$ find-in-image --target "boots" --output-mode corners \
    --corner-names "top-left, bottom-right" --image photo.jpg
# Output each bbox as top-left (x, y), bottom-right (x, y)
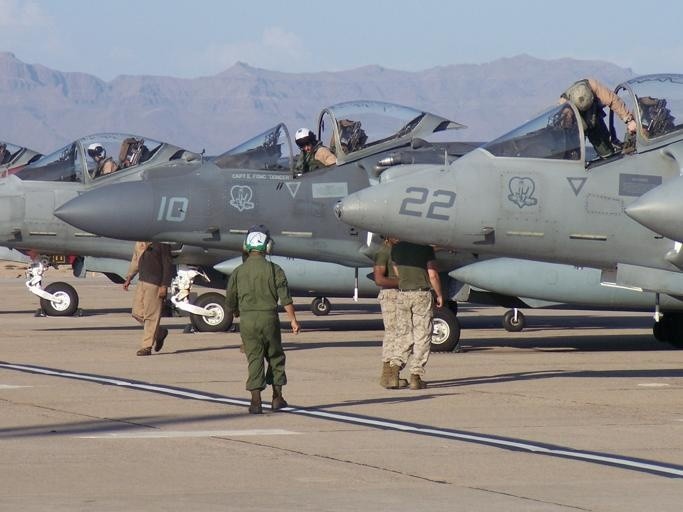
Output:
top-left (380, 363), bottom-right (426, 390)
top-left (249, 390), bottom-right (262, 414)
top-left (272, 385), bottom-right (287, 410)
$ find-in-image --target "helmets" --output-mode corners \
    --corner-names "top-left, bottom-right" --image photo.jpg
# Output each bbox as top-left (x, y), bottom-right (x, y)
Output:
top-left (294, 127), bottom-right (316, 148)
top-left (567, 83), bottom-right (594, 112)
top-left (88, 143), bottom-right (106, 159)
top-left (244, 225), bottom-right (270, 253)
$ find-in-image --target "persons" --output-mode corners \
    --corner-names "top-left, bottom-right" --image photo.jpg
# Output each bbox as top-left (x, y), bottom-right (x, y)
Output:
top-left (224, 232), bottom-right (301, 414)
top-left (386, 240), bottom-right (444, 390)
top-left (88, 142), bottom-right (120, 179)
top-left (374, 234), bottom-right (409, 389)
top-left (233, 224), bottom-right (276, 386)
top-left (560, 78), bottom-right (637, 161)
top-left (293, 127), bottom-right (337, 178)
top-left (124, 242), bottom-right (174, 356)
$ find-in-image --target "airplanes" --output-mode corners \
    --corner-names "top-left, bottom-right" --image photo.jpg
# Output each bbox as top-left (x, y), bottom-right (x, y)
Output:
top-left (0, 141), bottom-right (82, 318)
top-left (52, 97), bottom-right (527, 351)
top-left (624, 176), bottom-right (682, 270)
top-left (0, 133), bottom-right (368, 333)
top-left (333, 73), bottom-right (683, 355)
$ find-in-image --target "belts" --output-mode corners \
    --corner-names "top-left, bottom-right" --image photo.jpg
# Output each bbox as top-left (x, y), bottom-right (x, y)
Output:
top-left (399, 287), bottom-right (429, 292)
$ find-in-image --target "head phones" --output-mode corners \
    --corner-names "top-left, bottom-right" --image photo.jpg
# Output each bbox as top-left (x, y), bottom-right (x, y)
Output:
top-left (242, 240), bottom-right (273, 254)
top-left (95, 146), bottom-right (105, 153)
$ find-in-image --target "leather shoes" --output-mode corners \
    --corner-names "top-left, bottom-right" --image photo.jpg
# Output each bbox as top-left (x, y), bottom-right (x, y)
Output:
top-left (136, 348), bottom-right (151, 356)
top-left (154, 328), bottom-right (168, 351)
